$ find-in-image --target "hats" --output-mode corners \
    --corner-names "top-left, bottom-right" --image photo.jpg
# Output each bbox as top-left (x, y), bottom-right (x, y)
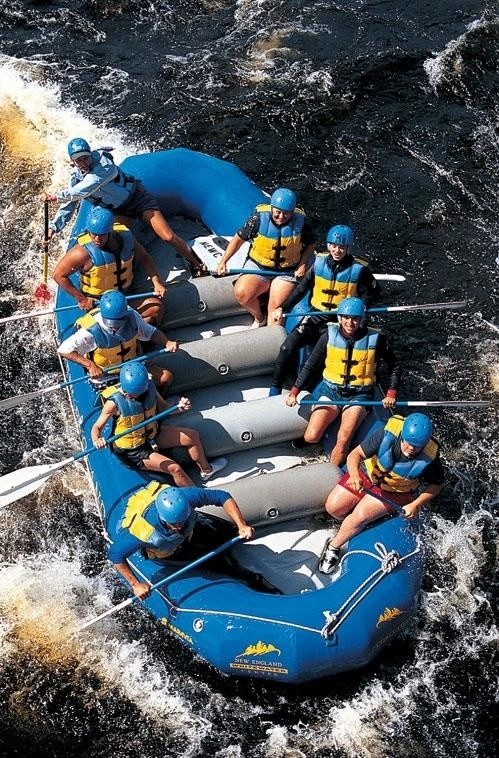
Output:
top-left (103, 316), bottom-right (126, 328)
top-left (70, 151), bottom-right (91, 160)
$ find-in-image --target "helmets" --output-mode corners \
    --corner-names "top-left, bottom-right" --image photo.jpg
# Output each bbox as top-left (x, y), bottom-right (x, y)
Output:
top-left (100, 291), bottom-right (128, 320)
top-left (68, 138), bottom-right (90, 158)
top-left (86, 208), bottom-right (113, 235)
top-left (399, 413), bottom-right (432, 448)
top-left (155, 486), bottom-right (191, 523)
top-left (326, 224), bottom-right (355, 255)
top-left (337, 297), bottom-right (367, 325)
top-left (119, 362), bottom-right (149, 395)
top-left (271, 188), bottom-right (296, 214)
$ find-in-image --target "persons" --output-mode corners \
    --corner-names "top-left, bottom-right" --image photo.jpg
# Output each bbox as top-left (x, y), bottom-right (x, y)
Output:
top-left (57, 290), bottom-right (178, 398)
top-left (287, 298), bottom-right (402, 466)
top-left (40, 138), bottom-right (207, 276)
top-left (107, 480), bottom-right (284, 602)
top-left (269, 225), bottom-right (382, 395)
top-left (216, 189), bottom-right (317, 329)
top-left (91, 362), bottom-right (227, 488)
top-left (319, 412), bottom-right (445, 574)
top-left (53, 209), bottom-right (168, 327)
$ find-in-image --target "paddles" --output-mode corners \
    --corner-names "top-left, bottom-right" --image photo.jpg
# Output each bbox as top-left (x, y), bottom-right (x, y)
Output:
top-left (281, 301), bottom-right (467, 317)
top-left (0, 400), bottom-right (186, 508)
top-left (210, 269), bottom-right (406, 282)
top-left (0, 292), bottom-right (156, 325)
top-left (33, 200), bottom-right (50, 301)
top-left (79, 532), bottom-right (246, 631)
top-left (0, 347), bottom-right (169, 412)
top-left (297, 401), bottom-right (490, 408)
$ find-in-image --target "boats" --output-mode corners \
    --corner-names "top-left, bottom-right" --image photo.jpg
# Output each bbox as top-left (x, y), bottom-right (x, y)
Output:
top-left (54, 147), bottom-right (425, 686)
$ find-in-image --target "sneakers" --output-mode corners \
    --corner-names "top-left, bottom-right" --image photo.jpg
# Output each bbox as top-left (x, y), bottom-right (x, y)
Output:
top-left (199, 458), bottom-right (228, 481)
top-left (319, 537), bottom-right (340, 575)
top-left (313, 511), bottom-right (342, 525)
top-left (247, 573), bottom-right (276, 594)
top-left (291, 437), bottom-right (307, 449)
top-left (249, 315), bottom-right (267, 329)
